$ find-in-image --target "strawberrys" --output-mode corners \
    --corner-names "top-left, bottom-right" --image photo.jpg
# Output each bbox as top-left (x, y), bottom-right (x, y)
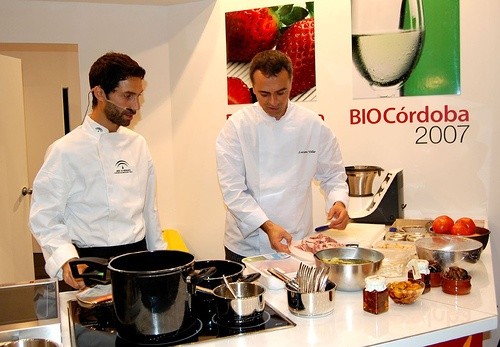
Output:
top-left (225, 1), bottom-right (316, 105)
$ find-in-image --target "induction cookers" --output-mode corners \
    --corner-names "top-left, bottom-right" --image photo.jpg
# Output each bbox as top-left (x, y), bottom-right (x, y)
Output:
top-left (59, 272), bottom-right (296, 346)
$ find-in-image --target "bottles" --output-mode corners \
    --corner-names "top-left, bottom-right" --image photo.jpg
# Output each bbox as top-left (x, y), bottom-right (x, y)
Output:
top-left (363, 275), bottom-right (389, 314)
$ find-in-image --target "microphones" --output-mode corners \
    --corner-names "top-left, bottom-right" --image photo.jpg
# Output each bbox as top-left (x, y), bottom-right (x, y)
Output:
top-left (105, 99), bottom-right (133, 112)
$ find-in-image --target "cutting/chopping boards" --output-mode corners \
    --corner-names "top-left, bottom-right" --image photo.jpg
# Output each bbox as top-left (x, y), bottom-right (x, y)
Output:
top-left (372, 218), bottom-right (484, 248)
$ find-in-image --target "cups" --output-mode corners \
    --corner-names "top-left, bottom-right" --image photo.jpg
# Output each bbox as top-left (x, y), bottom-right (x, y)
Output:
top-left (441, 274), bottom-right (471, 295)
top-left (408, 260), bottom-right (431, 295)
top-left (425, 270), bottom-right (446, 288)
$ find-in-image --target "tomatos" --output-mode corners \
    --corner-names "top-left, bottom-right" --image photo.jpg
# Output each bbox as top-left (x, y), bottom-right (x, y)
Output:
top-left (432, 216), bottom-right (479, 237)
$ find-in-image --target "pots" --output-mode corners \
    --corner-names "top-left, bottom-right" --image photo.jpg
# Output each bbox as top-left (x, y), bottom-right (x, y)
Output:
top-left (69, 250), bottom-right (195, 342)
top-left (194, 260), bottom-right (244, 289)
top-left (313, 244), bottom-right (384, 292)
top-left (194, 282), bottom-right (266, 324)
top-left (344, 166), bottom-right (384, 197)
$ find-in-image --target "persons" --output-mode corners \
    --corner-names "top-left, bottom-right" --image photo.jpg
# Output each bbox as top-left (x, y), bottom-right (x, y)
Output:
top-left (28, 52), bottom-right (167, 292)
top-left (215, 51), bottom-right (349, 268)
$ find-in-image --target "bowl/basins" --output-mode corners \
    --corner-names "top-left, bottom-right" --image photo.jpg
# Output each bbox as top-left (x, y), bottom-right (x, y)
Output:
top-left (428, 226), bottom-right (491, 249)
top-left (416, 237), bottom-right (483, 275)
top-left (387, 278), bottom-right (426, 305)
top-left (284, 277), bottom-right (336, 319)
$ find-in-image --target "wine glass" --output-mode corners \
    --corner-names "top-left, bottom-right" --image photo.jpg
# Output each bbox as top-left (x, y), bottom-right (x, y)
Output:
top-left (351, 0), bottom-right (425, 98)
